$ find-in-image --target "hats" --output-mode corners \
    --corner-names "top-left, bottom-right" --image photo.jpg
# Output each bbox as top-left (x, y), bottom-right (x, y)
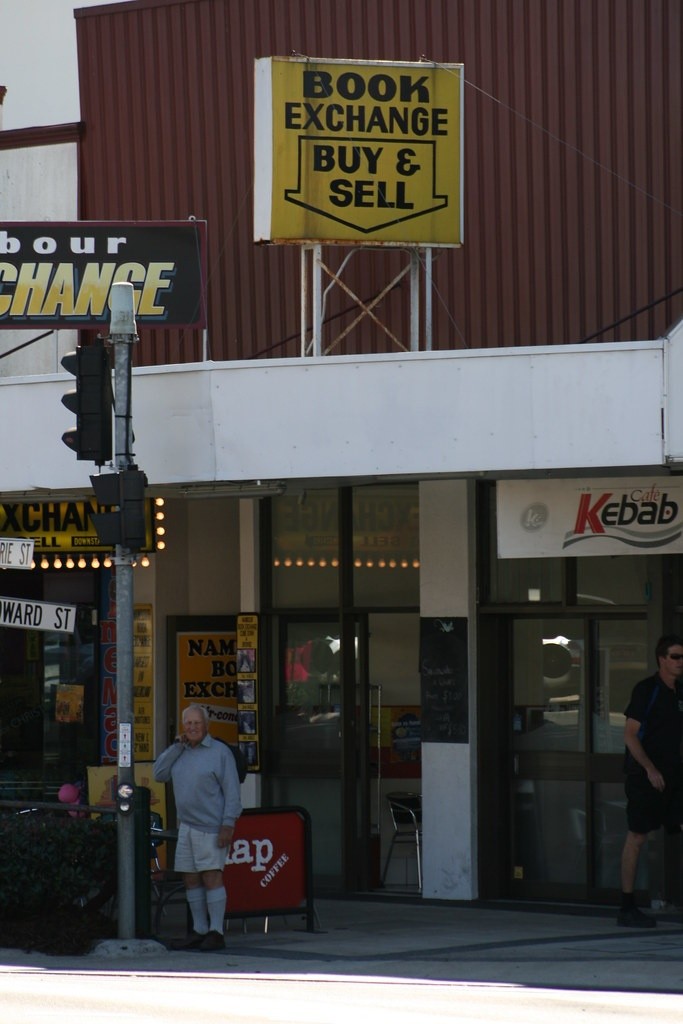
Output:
top-left (58, 783), bottom-right (81, 802)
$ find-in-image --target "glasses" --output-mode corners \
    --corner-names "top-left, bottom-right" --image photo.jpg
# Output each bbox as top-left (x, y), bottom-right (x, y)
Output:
top-left (670, 653), bottom-right (683, 660)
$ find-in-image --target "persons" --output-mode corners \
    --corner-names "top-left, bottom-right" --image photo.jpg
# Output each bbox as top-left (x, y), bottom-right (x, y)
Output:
top-left (617, 633), bottom-right (683, 927)
top-left (153, 703), bottom-right (242, 951)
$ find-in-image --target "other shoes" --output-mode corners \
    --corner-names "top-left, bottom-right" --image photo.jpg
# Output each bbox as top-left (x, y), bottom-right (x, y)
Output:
top-left (198, 930), bottom-right (226, 951)
top-left (617, 907), bottom-right (657, 927)
top-left (171, 938), bottom-right (199, 949)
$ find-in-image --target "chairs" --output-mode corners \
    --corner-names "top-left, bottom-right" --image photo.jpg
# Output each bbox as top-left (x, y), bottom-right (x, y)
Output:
top-left (380, 791), bottom-right (422, 892)
top-left (98, 809), bottom-right (167, 920)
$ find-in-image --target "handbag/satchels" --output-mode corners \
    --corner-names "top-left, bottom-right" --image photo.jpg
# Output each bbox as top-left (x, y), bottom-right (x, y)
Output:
top-left (625, 684), bottom-right (660, 762)
